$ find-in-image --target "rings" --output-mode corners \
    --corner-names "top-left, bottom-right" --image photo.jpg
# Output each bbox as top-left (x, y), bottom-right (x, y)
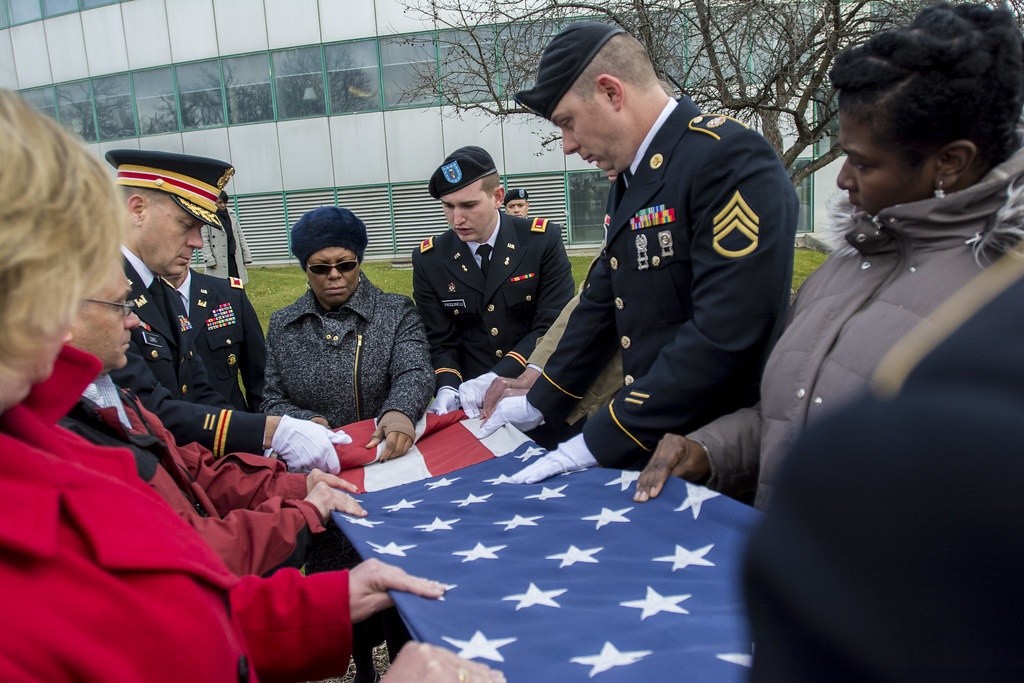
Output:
top-left (458, 666), bottom-right (472, 683)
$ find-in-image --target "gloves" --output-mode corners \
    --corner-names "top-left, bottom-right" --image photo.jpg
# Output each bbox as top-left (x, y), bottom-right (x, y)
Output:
top-left (458, 371), bottom-right (499, 419)
top-left (427, 386), bottom-right (463, 416)
top-left (475, 395), bottom-right (543, 439)
top-left (271, 415), bottom-right (353, 474)
top-left (509, 432), bottom-right (599, 486)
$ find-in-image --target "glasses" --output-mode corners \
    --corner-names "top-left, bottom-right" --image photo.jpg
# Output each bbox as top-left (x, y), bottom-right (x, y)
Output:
top-left (307, 254), bottom-right (358, 276)
top-left (85, 298), bottom-right (135, 317)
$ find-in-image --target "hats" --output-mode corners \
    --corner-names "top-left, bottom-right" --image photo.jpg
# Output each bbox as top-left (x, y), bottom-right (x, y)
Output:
top-left (512, 21), bottom-right (627, 119)
top-left (105, 149), bottom-right (236, 231)
top-left (503, 188), bottom-right (528, 206)
top-left (428, 145), bottom-right (498, 199)
top-left (291, 205), bottom-right (369, 271)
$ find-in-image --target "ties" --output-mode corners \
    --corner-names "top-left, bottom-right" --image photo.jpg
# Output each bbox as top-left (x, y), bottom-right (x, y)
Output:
top-left (614, 166), bottom-right (634, 218)
top-left (173, 291), bottom-right (189, 323)
top-left (475, 244), bottom-right (492, 277)
top-left (148, 278), bottom-right (171, 332)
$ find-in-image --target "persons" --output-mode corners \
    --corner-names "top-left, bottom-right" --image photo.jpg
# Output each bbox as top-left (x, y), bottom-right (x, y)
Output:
top-left (478, 21), bottom-right (801, 484)
top-left (412, 144), bottom-right (574, 420)
top-left (481, 162), bottom-right (625, 453)
top-left (637, 4), bottom-right (1024, 683)
top-left (0, 86), bottom-right (507, 683)
top-left (502, 188), bottom-right (529, 219)
